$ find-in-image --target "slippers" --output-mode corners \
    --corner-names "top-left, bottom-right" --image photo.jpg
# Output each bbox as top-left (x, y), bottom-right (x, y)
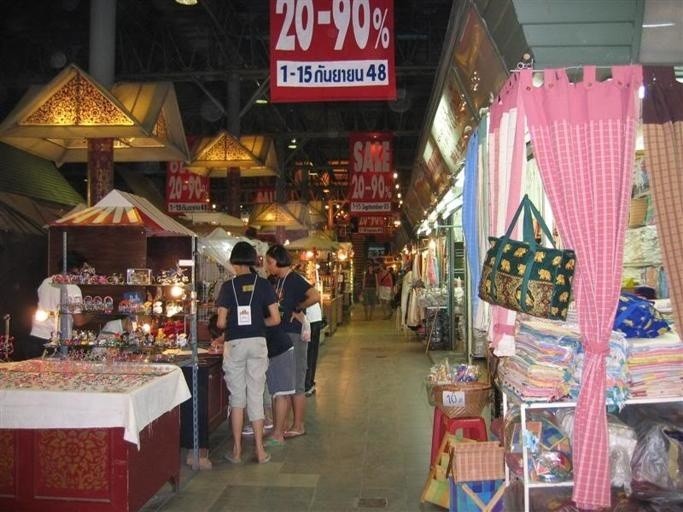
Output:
top-left (261, 433), bottom-right (286, 447)
top-left (257, 450), bottom-right (271, 464)
top-left (222, 450), bottom-right (243, 465)
top-left (283, 428), bottom-right (305, 438)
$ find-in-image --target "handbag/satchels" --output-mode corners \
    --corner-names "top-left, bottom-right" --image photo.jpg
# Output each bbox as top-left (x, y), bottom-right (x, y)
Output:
top-left (612, 292), bottom-right (672, 339)
top-left (477, 235), bottom-right (577, 321)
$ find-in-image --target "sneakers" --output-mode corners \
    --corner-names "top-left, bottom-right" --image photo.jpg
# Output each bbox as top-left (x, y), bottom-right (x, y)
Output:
top-left (305, 384), bottom-right (315, 397)
top-left (261, 416), bottom-right (274, 428)
top-left (241, 423), bottom-right (265, 435)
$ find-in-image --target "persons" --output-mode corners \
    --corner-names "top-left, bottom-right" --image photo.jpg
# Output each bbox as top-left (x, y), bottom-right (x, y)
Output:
top-left (95, 313), bottom-right (136, 341)
top-left (360, 262), bottom-right (405, 322)
top-left (217, 241), bottom-right (280, 464)
top-left (209, 266), bottom-right (322, 449)
top-left (266, 246), bottom-right (320, 439)
top-left (29, 250), bottom-right (91, 360)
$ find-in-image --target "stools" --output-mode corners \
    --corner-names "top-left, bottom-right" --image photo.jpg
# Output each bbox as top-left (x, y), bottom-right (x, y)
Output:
top-left (439, 413), bottom-right (489, 452)
top-left (432, 406), bottom-right (447, 463)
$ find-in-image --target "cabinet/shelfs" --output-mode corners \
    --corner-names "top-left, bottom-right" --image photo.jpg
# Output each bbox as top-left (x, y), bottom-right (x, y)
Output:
top-left (50, 262), bottom-right (196, 367)
top-left (0, 359), bottom-right (192, 511)
top-left (180, 354), bottom-right (231, 452)
top-left (502, 389), bottom-right (683, 512)
